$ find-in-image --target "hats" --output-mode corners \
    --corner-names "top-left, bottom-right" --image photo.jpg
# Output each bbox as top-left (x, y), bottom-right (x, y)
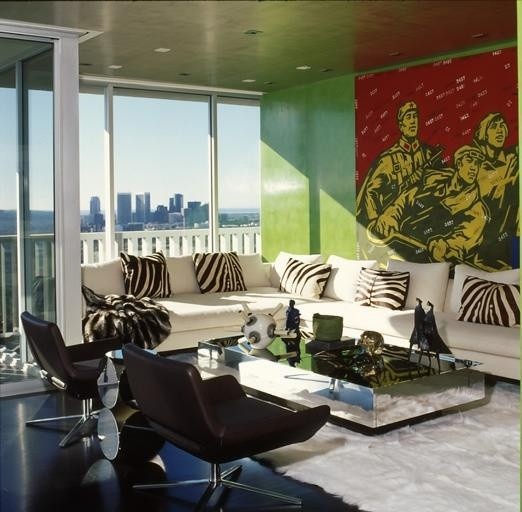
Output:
top-left (454, 145), bottom-right (482, 164)
top-left (398, 101), bottom-right (417, 121)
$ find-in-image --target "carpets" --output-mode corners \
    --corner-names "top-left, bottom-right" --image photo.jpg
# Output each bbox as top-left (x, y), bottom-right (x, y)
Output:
top-left (161, 345), bottom-right (521, 511)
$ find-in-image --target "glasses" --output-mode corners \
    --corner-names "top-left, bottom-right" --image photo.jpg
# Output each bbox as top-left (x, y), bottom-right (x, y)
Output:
top-left (459, 150), bottom-right (486, 161)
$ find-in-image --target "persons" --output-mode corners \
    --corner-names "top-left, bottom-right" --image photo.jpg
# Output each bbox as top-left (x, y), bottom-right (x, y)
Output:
top-left (356, 101), bottom-right (448, 260)
top-left (375, 144), bottom-right (492, 279)
top-left (471, 112), bottom-right (519, 272)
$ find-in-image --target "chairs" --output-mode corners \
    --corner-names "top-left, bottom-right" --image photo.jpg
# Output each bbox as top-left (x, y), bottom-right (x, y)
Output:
top-left (121, 342), bottom-right (331, 512)
top-left (21, 311), bottom-right (124, 448)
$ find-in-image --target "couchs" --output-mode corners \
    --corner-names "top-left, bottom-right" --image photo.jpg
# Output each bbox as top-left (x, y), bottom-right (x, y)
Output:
top-left (77, 251), bottom-right (522, 383)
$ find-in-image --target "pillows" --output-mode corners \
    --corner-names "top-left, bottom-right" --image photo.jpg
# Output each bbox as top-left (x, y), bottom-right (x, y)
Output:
top-left (237, 253), bottom-right (272, 287)
top-left (192, 252), bottom-right (248, 294)
top-left (324, 255), bottom-right (378, 303)
top-left (449, 264), bottom-right (520, 313)
top-left (166, 256), bottom-right (201, 295)
top-left (269, 251), bottom-right (322, 288)
top-left (81, 257), bottom-right (125, 295)
top-left (353, 267), bottom-right (409, 310)
top-left (457, 274), bottom-right (520, 328)
top-left (386, 259), bottom-right (452, 312)
top-left (120, 250), bottom-right (171, 298)
top-left (277, 257), bottom-right (332, 299)
